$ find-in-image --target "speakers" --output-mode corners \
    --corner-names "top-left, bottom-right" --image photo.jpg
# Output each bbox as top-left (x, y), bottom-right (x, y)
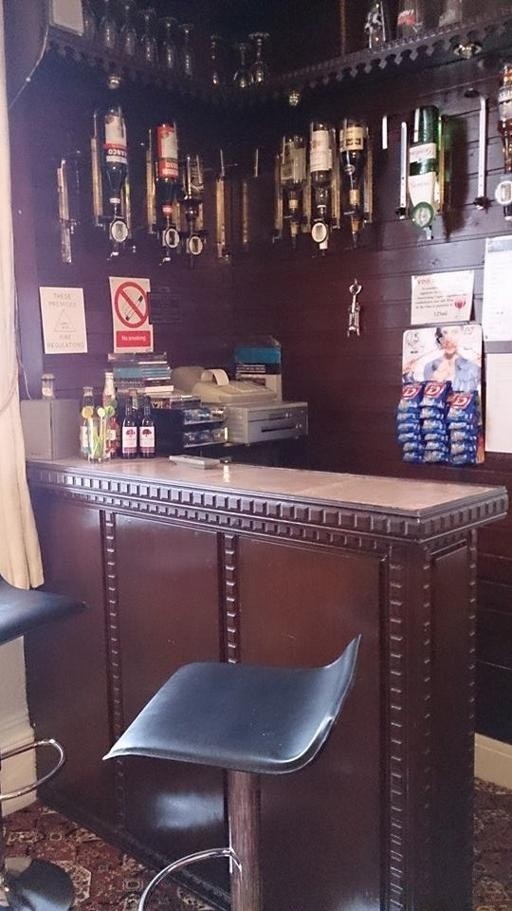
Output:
top-left (21, 398), bottom-right (81, 460)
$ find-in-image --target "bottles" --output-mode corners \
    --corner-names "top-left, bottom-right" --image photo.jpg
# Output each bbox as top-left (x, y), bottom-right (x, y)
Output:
top-left (41, 373), bottom-right (55, 400)
top-left (181, 147), bottom-right (203, 259)
top-left (149, 109), bottom-right (181, 263)
top-left (308, 117), bottom-right (331, 257)
top-left (498, 65), bottom-right (512, 222)
top-left (93, 105), bottom-right (128, 260)
top-left (280, 131), bottom-right (304, 255)
top-left (338, 115), bottom-right (365, 250)
top-left (408, 105), bottom-right (440, 242)
top-left (81, 372), bottom-right (155, 459)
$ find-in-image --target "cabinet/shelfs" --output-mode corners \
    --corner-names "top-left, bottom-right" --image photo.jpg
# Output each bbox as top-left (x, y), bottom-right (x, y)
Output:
top-left (22, 453), bottom-right (509, 911)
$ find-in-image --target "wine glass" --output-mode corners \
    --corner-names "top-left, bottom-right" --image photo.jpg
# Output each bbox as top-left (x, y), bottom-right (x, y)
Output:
top-left (80, 0), bottom-right (269, 83)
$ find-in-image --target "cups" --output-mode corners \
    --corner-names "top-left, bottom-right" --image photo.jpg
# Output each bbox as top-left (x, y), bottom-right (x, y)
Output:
top-left (356, 1), bottom-right (463, 49)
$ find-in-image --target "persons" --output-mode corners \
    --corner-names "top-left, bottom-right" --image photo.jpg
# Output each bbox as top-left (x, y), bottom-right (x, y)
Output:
top-left (422, 327), bottom-right (482, 396)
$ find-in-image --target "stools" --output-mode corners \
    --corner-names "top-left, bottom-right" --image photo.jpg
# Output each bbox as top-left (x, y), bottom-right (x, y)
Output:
top-left (100, 633), bottom-right (361, 911)
top-left (1, 565), bottom-right (91, 911)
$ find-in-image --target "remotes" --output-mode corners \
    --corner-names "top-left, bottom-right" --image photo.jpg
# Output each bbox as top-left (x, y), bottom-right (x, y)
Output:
top-left (169, 454), bottom-right (220, 469)
